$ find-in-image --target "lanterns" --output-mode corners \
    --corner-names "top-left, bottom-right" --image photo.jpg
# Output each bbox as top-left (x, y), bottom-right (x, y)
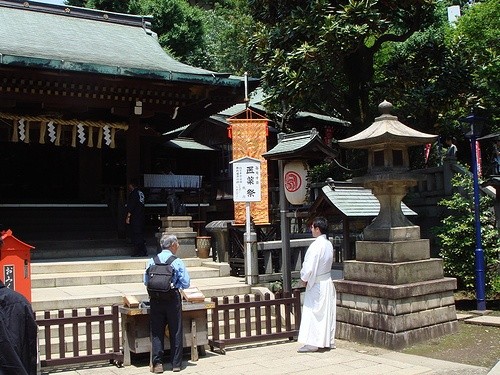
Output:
top-left (283, 160), bottom-right (310, 208)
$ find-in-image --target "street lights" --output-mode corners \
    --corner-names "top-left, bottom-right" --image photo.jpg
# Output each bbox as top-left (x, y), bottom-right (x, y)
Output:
top-left (457, 112), bottom-right (489, 312)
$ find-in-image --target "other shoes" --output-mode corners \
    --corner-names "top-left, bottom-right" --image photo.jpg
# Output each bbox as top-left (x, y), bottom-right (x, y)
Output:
top-left (297, 345), bottom-right (318, 352)
top-left (150, 363), bottom-right (163, 373)
top-left (173, 367), bottom-right (181, 372)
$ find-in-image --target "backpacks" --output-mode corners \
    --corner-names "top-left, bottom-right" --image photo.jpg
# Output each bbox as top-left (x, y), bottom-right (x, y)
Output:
top-left (146, 254), bottom-right (177, 299)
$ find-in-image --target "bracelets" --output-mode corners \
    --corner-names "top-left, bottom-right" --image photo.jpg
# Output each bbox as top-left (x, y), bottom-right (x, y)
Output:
top-left (127, 216), bottom-right (130, 218)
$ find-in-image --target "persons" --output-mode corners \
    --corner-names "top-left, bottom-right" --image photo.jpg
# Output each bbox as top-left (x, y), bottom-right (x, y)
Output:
top-left (445, 137), bottom-right (457, 158)
top-left (125, 181), bottom-right (148, 256)
top-left (0, 279), bottom-right (38, 375)
top-left (143, 234), bottom-right (190, 373)
top-left (296, 217), bottom-right (336, 352)
